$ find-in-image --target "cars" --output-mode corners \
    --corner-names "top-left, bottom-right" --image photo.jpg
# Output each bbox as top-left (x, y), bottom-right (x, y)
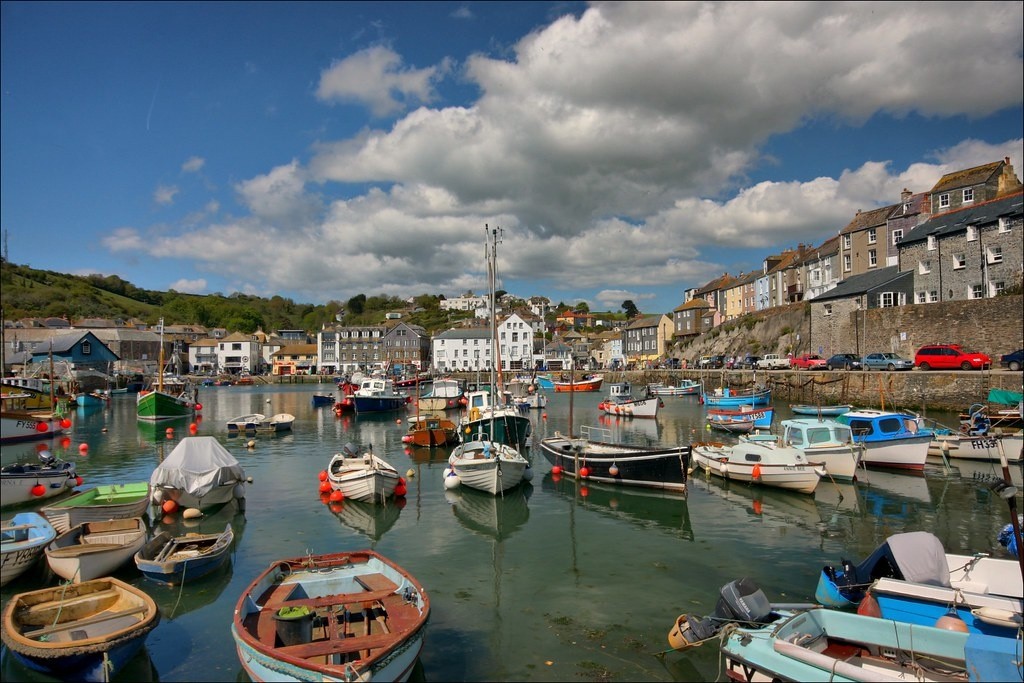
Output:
top-left (663, 343), bottom-right (1024, 372)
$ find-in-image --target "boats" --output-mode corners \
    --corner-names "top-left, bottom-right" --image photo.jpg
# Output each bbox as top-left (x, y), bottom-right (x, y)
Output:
top-left (254, 413), bottom-right (295, 432)
top-left (667, 529), bottom-right (1024, 682)
top-left (40, 481), bottom-right (151, 534)
top-left (0, 576), bottom-right (161, 683)
top-left (0, 360), bottom-right (78, 410)
top-left (94, 317), bottom-right (254, 420)
top-left (604, 414), bottom-right (664, 440)
top-left (132, 560), bottom-right (233, 621)
top-left (227, 414), bottom-right (267, 433)
top-left (0, 450), bottom-right (77, 507)
top-left (137, 420), bottom-right (190, 449)
top-left (1, 512), bottom-right (57, 586)
top-left (135, 522), bottom-right (234, 586)
top-left (151, 436), bottom-right (244, 509)
top-left (0, 393), bottom-right (65, 446)
top-left (230, 549), bottom-right (431, 683)
top-left (311, 374), bottom-right (1024, 505)
top-left (325, 454), bottom-right (1023, 542)
top-left (44, 517), bottom-right (149, 584)
top-left (76, 393), bottom-right (108, 407)
